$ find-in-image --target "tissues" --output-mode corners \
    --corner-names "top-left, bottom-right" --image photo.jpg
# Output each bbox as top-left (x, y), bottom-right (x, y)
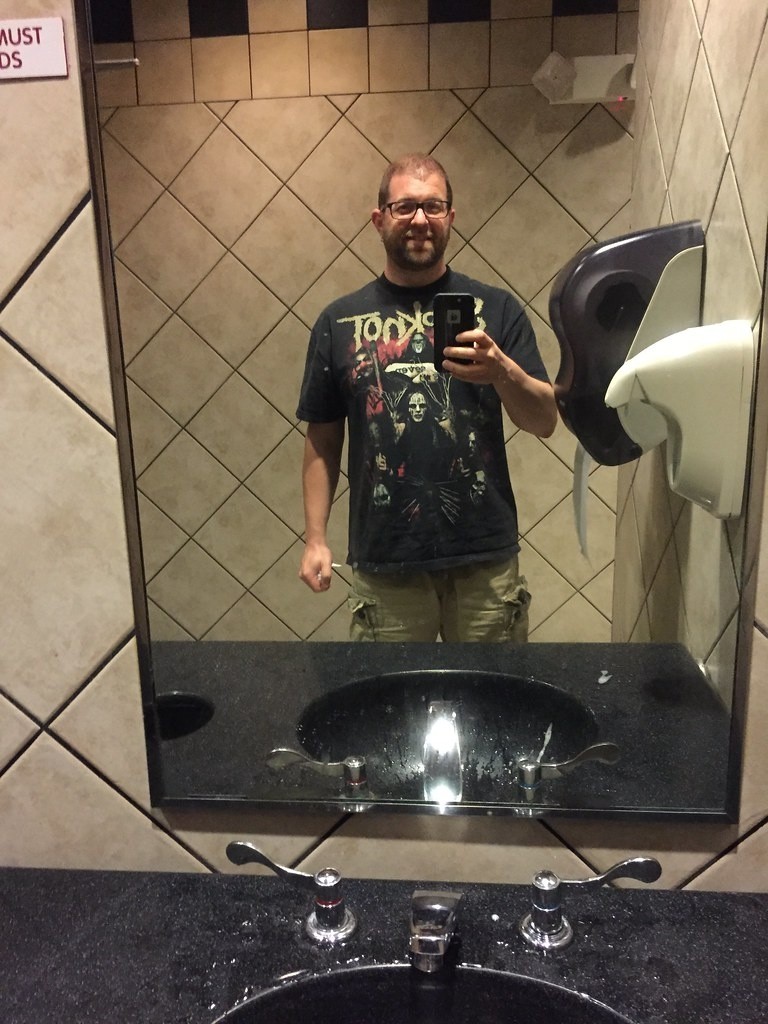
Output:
top-left (547, 221), bottom-right (704, 551)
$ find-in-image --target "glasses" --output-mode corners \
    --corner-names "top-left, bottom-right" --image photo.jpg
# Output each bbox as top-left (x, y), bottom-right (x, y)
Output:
top-left (381, 198), bottom-right (451, 220)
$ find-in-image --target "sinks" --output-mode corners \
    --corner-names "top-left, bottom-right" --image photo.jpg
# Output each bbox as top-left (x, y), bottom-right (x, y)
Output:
top-left (301, 666), bottom-right (612, 779)
top-left (205, 964), bottom-right (632, 1024)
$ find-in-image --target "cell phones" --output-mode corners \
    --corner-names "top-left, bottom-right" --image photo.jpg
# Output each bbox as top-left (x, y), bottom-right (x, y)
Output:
top-left (432, 291), bottom-right (477, 373)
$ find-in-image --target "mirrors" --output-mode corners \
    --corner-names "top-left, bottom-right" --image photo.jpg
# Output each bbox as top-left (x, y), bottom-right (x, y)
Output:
top-left (73, 0), bottom-right (768, 826)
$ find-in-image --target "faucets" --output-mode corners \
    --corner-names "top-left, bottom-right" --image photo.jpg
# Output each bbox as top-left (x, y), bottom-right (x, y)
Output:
top-left (422, 699), bottom-right (472, 808)
top-left (406, 887), bottom-right (466, 971)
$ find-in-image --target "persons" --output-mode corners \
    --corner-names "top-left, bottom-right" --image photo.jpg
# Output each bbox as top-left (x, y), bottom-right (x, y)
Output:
top-left (293, 148), bottom-right (559, 642)
